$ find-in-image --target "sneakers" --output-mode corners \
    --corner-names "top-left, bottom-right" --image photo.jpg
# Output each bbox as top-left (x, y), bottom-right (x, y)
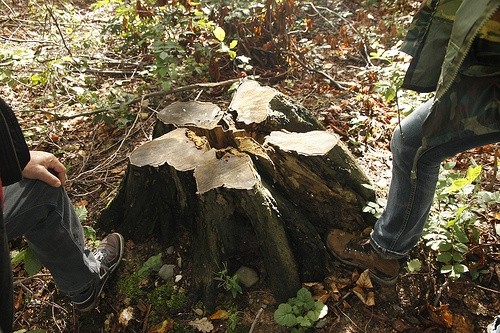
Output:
top-left (68, 232), bottom-right (124, 311)
top-left (326, 229), bottom-right (400, 287)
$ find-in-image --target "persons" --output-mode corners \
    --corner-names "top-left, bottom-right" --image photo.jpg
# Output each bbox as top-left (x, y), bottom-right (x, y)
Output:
top-left (0, 96), bottom-right (124, 333)
top-left (326, 0), bottom-right (500, 286)
